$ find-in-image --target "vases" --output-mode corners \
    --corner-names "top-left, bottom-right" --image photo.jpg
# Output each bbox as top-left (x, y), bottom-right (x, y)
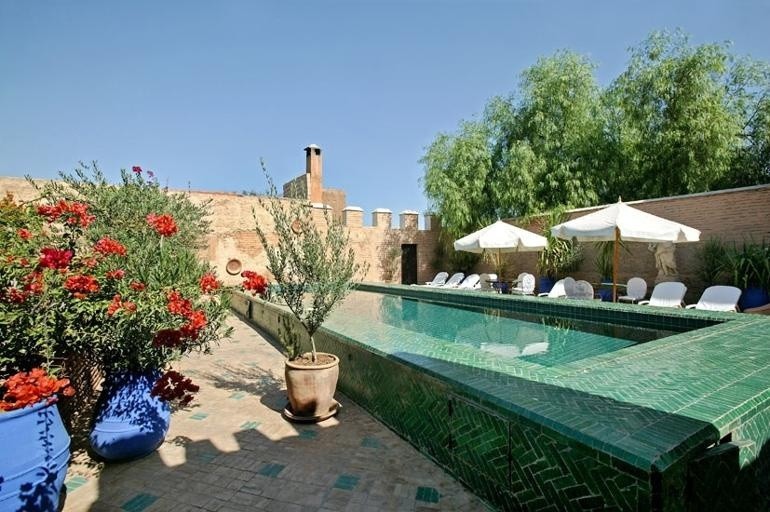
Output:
top-left (88, 361), bottom-right (170, 464)
top-left (0, 394), bottom-right (72, 512)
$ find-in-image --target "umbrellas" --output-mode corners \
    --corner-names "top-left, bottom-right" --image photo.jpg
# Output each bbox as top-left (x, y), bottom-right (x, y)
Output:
top-left (550, 194), bottom-right (703, 303)
top-left (452, 216), bottom-right (550, 282)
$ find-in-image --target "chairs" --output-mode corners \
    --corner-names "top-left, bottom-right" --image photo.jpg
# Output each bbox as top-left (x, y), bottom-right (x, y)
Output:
top-left (617, 277), bottom-right (649, 304)
top-left (510, 273), bottom-right (535, 296)
top-left (411, 272), bottom-right (497, 291)
top-left (685, 283), bottom-right (741, 313)
top-left (537, 277), bottom-right (598, 300)
top-left (638, 281), bottom-right (688, 311)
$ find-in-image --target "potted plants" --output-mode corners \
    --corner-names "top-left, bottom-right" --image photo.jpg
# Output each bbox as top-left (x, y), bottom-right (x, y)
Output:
top-left (493, 251), bottom-right (509, 293)
top-left (591, 241), bottom-right (631, 301)
top-left (536, 204), bottom-right (585, 296)
top-left (250, 158), bottom-right (368, 425)
top-left (737, 232), bottom-right (770, 312)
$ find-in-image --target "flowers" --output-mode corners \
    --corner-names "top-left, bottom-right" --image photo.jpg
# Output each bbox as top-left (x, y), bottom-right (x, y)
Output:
top-left (0, 160), bottom-right (267, 413)
